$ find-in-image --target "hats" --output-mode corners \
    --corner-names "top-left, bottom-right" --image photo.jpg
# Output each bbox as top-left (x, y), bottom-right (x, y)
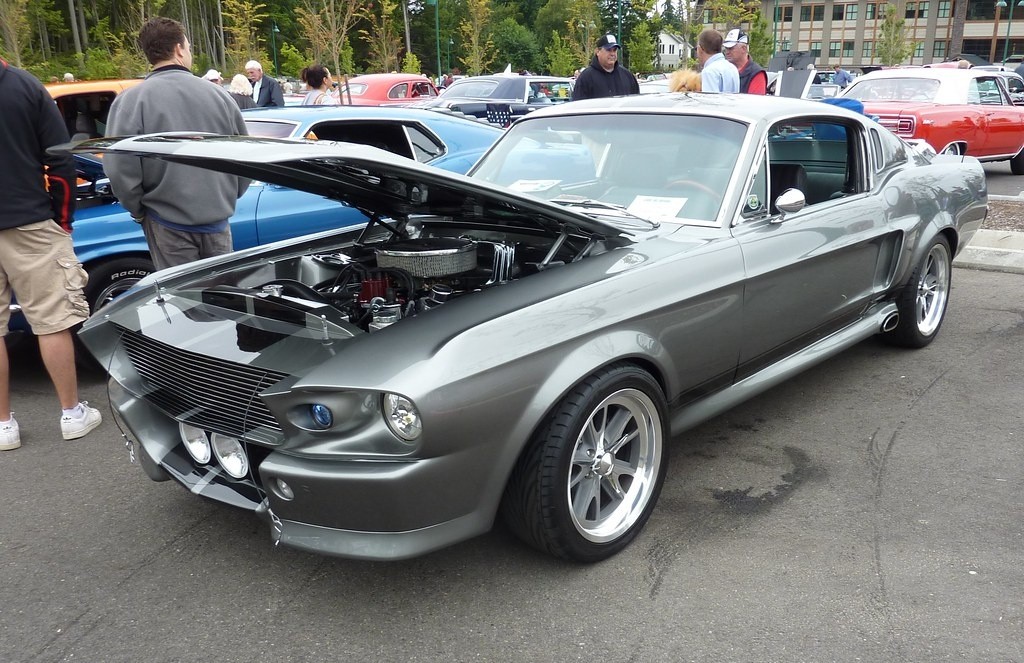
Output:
top-left (206, 70), bottom-right (220, 80)
top-left (723, 29), bottom-right (750, 47)
top-left (63, 73), bottom-right (74, 79)
top-left (597, 35), bottom-right (621, 49)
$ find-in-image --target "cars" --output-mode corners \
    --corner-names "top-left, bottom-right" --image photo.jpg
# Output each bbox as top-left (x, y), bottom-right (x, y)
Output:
top-left (637, 79), bottom-right (670, 94)
top-left (330, 74), bottom-right (440, 108)
top-left (377, 76), bottom-right (575, 127)
top-left (41, 80), bottom-right (145, 185)
top-left (8, 104), bottom-right (595, 352)
top-left (817, 71), bottom-right (837, 85)
top-left (775, 70), bottom-right (841, 101)
top-left (777, 68), bottom-right (1024, 175)
top-left (435, 75), bottom-right (465, 86)
top-left (47, 89), bottom-right (988, 565)
top-left (971, 65), bottom-right (1006, 86)
top-left (967, 73), bottom-right (1024, 106)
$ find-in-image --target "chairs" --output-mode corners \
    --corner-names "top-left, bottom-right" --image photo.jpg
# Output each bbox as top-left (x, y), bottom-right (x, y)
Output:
top-left (71, 114), bottom-right (101, 143)
top-left (769, 164), bottom-right (807, 216)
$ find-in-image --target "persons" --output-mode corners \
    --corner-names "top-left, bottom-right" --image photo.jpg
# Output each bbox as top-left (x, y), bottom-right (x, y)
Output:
top-left (807, 64), bottom-right (825, 99)
top-left (103, 17), bottom-right (252, 271)
top-left (227, 74), bottom-right (260, 110)
top-left (518, 69), bottom-right (532, 76)
top-left (51, 76), bottom-right (60, 84)
top-left (302, 65), bottom-right (340, 105)
top-left (63, 73), bottom-right (73, 83)
top-left (0, 56), bottom-right (101, 450)
top-left (696, 29), bottom-right (739, 92)
top-left (441, 73), bottom-right (453, 86)
top-left (572, 34), bottom-right (640, 102)
top-left (284, 79), bottom-right (292, 94)
top-left (201, 69), bottom-right (224, 87)
top-left (1012, 57), bottom-right (1024, 90)
top-left (723, 29), bottom-right (768, 95)
top-left (393, 74), bottom-right (434, 98)
top-left (832, 64), bottom-right (853, 89)
top-left (540, 85), bottom-right (549, 97)
top-left (957, 60), bottom-right (968, 69)
top-left (245, 61), bottom-right (285, 109)
top-left (574, 67), bottom-right (586, 79)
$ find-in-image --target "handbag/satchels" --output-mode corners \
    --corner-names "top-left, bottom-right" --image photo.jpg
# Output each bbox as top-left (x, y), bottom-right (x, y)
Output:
top-left (487, 103), bottom-right (513, 128)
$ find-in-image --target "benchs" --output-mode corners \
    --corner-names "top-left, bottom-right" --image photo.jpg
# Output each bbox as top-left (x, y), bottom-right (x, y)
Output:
top-left (803, 167), bottom-right (847, 206)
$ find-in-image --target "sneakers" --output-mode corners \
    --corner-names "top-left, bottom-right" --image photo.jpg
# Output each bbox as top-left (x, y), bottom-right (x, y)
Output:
top-left (0, 412), bottom-right (21, 450)
top-left (60, 401), bottom-right (102, 440)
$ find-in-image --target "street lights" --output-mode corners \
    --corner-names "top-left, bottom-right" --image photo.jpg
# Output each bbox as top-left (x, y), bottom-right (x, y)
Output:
top-left (447, 34), bottom-right (454, 73)
top-left (427, 0), bottom-right (441, 85)
top-left (272, 20), bottom-right (280, 73)
top-left (996, 0), bottom-right (1024, 66)
top-left (578, 20), bottom-right (596, 66)
